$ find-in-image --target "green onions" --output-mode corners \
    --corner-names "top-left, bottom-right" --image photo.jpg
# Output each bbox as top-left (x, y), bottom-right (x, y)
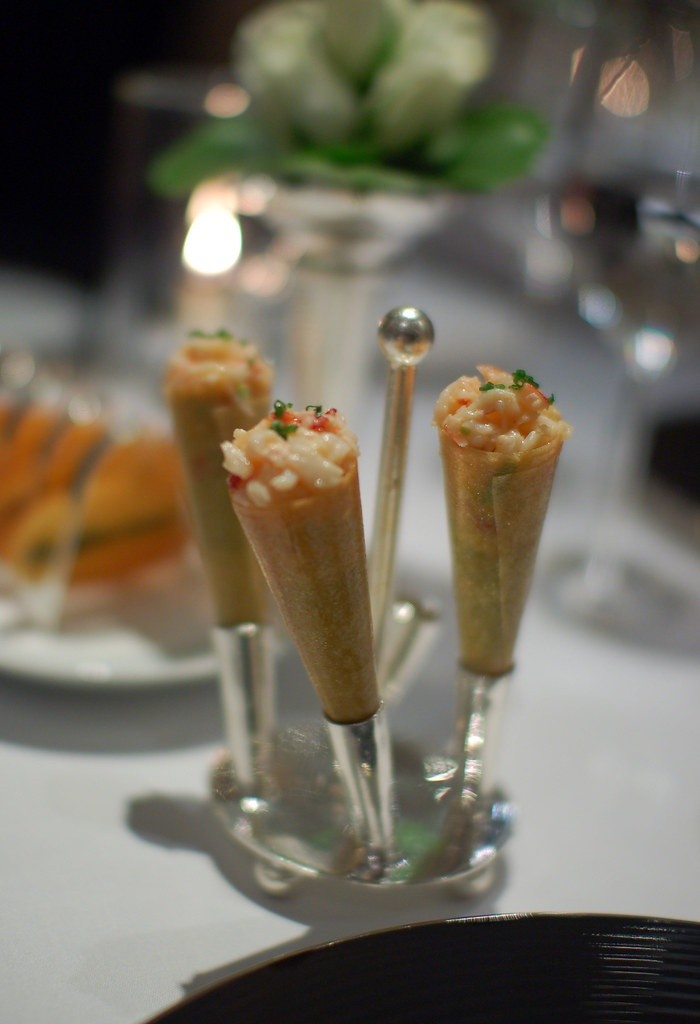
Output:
top-left (190, 328), bottom-right (555, 441)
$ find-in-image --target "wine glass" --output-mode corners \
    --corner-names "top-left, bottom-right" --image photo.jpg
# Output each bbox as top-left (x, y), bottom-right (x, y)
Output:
top-left (511, 18), bottom-right (700, 663)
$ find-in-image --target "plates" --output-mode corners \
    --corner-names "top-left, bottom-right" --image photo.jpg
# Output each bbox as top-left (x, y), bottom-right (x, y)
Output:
top-left (1, 585), bottom-right (286, 685)
top-left (145, 914), bottom-right (700, 1024)
